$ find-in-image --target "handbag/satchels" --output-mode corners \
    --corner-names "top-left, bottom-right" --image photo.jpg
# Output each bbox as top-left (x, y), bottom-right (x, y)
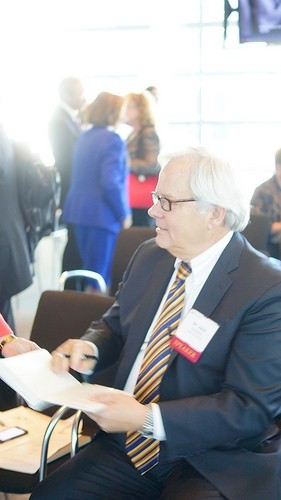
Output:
top-left (126, 173), bottom-right (158, 208)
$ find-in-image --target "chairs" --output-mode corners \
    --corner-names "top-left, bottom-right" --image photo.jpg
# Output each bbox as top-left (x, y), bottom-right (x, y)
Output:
top-left (0, 289), bottom-right (118, 483)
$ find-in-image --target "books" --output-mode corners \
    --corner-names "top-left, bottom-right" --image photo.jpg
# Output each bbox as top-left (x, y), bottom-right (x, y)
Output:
top-left (0, 348), bottom-right (136, 413)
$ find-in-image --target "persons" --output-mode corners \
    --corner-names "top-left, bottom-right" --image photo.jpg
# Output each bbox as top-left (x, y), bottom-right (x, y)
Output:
top-left (57, 92), bottom-right (133, 296)
top-left (249, 148), bottom-right (281, 260)
top-left (0, 312), bottom-right (42, 359)
top-left (0, 117), bottom-right (33, 336)
top-left (119, 86), bottom-right (161, 229)
top-left (46, 76), bottom-right (108, 293)
top-left (50, 148), bottom-right (281, 500)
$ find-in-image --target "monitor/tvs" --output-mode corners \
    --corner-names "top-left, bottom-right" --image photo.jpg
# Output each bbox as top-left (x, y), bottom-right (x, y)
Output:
top-left (238, 0), bottom-right (281, 44)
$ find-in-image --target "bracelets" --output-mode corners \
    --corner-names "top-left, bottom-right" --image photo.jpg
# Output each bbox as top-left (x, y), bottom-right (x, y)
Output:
top-left (0, 332), bottom-right (15, 354)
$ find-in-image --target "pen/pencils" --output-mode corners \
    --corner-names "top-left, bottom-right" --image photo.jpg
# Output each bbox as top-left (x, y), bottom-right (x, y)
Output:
top-left (58, 353), bottom-right (99, 360)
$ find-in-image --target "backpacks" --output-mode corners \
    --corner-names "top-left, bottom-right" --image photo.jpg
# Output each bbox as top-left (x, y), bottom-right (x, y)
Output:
top-left (12, 142), bottom-right (56, 263)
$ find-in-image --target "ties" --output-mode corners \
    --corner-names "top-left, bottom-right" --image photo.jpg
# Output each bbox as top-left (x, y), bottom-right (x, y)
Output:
top-left (125, 261), bottom-right (192, 477)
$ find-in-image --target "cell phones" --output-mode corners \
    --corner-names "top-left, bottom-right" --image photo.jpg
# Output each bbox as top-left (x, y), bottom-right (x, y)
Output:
top-left (0, 426), bottom-right (28, 443)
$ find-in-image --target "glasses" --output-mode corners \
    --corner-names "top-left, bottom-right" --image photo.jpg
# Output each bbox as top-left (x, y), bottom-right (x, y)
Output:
top-left (151, 192), bottom-right (198, 212)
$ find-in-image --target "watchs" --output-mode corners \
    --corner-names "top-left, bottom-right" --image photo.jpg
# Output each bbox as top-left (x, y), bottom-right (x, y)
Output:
top-left (140, 408), bottom-right (154, 438)
